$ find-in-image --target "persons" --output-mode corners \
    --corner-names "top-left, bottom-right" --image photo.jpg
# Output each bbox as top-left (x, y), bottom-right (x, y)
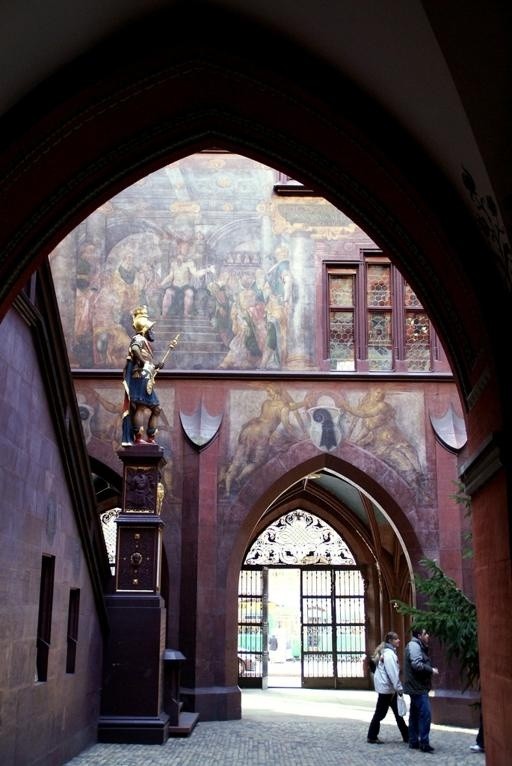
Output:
top-left (124, 311), bottom-right (168, 446)
top-left (404, 627), bottom-right (440, 752)
top-left (223, 382), bottom-right (311, 498)
top-left (468, 705), bottom-right (483, 752)
top-left (79, 195), bottom-right (293, 368)
top-left (367, 631), bottom-right (408, 746)
top-left (351, 383), bottom-right (422, 492)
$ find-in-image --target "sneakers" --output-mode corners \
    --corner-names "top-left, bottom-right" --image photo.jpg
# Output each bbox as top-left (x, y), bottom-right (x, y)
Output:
top-left (407, 742), bottom-right (435, 752)
top-left (469, 745), bottom-right (485, 753)
top-left (367, 737), bottom-right (385, 744)
